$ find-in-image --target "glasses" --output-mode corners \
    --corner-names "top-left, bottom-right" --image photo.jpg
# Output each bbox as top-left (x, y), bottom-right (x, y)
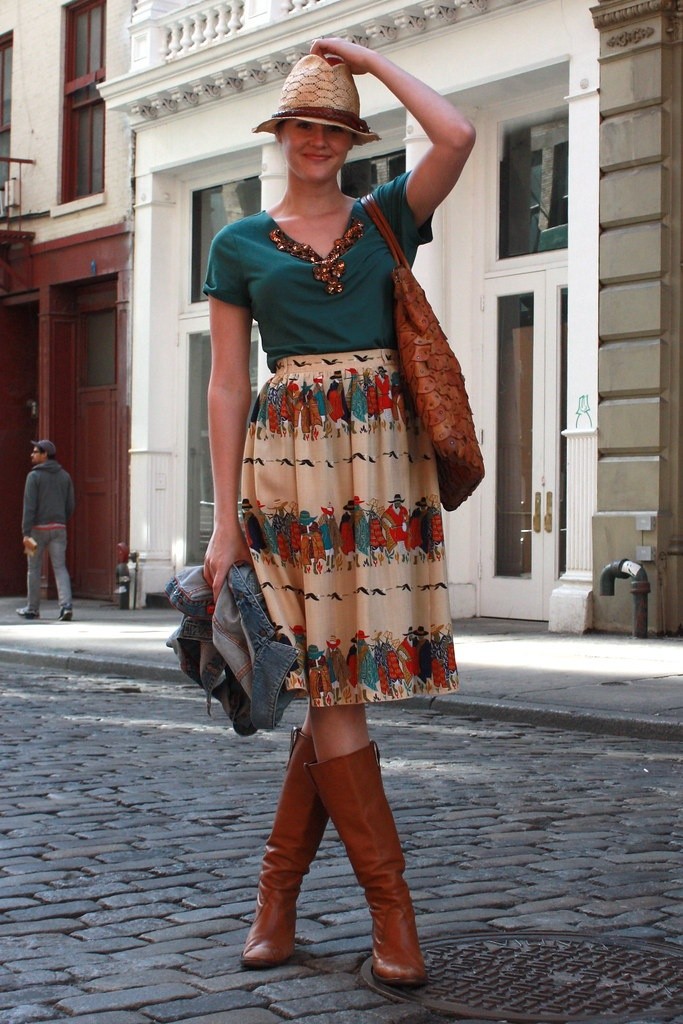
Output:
top-left (32, 450), bottom-right (39, 453)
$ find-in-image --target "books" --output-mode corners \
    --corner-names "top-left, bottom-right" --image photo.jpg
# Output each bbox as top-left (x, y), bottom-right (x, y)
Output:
top-left (24, 538), bottom-right (37, 557)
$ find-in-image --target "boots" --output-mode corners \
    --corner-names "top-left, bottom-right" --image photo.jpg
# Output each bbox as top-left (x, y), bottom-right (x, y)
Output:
top-left (241, 727), bottom-right (330, 968)
top-left (305, 738), bottom-right (430, 987)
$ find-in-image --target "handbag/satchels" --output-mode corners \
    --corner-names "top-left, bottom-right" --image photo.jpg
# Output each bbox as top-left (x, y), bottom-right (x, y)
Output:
top-left (362, 193), bottom-right (485, 512)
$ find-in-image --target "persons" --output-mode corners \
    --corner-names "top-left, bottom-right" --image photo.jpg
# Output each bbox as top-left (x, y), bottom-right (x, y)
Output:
top-left (15, 440), bottom-right (75, 621)
top-left (201, 37), bottom-right (475, 987)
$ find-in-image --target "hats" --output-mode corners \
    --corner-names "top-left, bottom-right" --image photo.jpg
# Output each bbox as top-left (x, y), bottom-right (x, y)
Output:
top-left (252, 55), bottom-right (383, 147)
top-left (31, 440), bottom-right (56, 456)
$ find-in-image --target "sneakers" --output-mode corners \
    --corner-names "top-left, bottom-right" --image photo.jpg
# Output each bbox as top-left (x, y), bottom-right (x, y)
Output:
top-left (16, 608), bottom-right (39, 619)
top-left (57, 607), bottom-right (72, 620)
